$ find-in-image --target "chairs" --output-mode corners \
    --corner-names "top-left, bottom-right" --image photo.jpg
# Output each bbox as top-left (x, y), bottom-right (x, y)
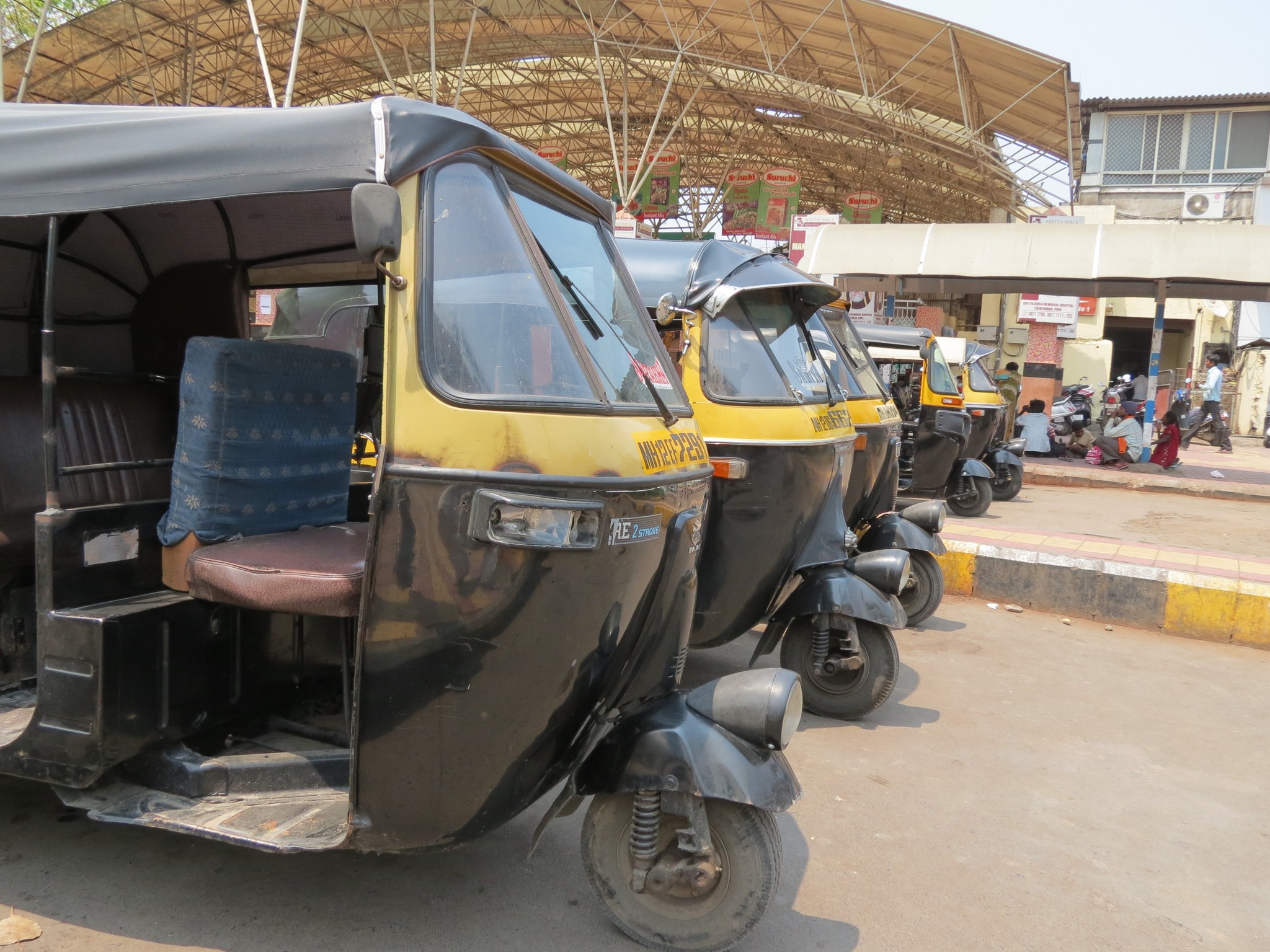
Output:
top-left (156, 335), bottom-right (370, 748)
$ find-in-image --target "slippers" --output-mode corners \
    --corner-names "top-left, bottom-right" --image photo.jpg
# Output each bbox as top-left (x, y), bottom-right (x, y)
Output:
top-left (1058, 455), bottom-right (1066, 461)
top-left (1168, 462), bottom-right (1183, 470)
top-left (1106, 462), bottom-right (1130, 469)
top-left (1066, 455), bottom-right (1074, 462)
top-left (1173, 458), bottom-right (1180, 465)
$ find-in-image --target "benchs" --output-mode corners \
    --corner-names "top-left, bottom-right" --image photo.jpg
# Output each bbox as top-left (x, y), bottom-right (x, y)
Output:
top-left (1, 370), bottom-right (180, 677)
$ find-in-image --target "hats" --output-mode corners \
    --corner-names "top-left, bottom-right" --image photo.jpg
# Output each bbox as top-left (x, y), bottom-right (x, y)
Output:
top-left (1121, 401), bottom-right (1138, 416)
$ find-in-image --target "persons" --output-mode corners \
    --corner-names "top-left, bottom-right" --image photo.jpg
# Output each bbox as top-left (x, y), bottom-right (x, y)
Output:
top-left (1064, 420), bottom-right (1095, 460)
top-left (992, 361), bottom-right (1022, 440)
top-left (1130, 370), bottom-right (1149, 403)
top-left (1043, 411), bottom-right (1056, 441)
top-left (1092, 399), bottom-right (1145, 471)
top-left (1012, 397), bottom-right (1052, 457)
top-left (1176, 352), bottom-right (1234, 453)
top-left (1148, 411), bottom-right (1181, 469)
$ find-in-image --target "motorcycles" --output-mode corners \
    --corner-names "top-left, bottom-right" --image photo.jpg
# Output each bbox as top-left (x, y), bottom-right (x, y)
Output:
top-left (0, 103), bottom-right (800, 950)
top-left (808, 296), bottom-right (948, 627)
top-left (867, 334), bottom-right (1024, 504)
top-left (853, 322), bottom-right (994, 516)
top-left (609, 235), bottom-right (909, 720)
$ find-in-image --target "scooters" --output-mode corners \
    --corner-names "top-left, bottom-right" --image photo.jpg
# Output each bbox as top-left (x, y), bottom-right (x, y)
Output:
top-left (1050, 375), bottom-right (1156, 438)
top-left (1162, 378), bottom-right (1228, 446)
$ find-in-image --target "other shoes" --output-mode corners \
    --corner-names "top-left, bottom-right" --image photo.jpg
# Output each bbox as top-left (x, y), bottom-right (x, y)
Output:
top-left (1066, 451), bottom-right (1082, 458)
top-left (1216, 449), bottom-right (1234, 454)
top-left (1178, 445), bottom-right (1188, 450)
